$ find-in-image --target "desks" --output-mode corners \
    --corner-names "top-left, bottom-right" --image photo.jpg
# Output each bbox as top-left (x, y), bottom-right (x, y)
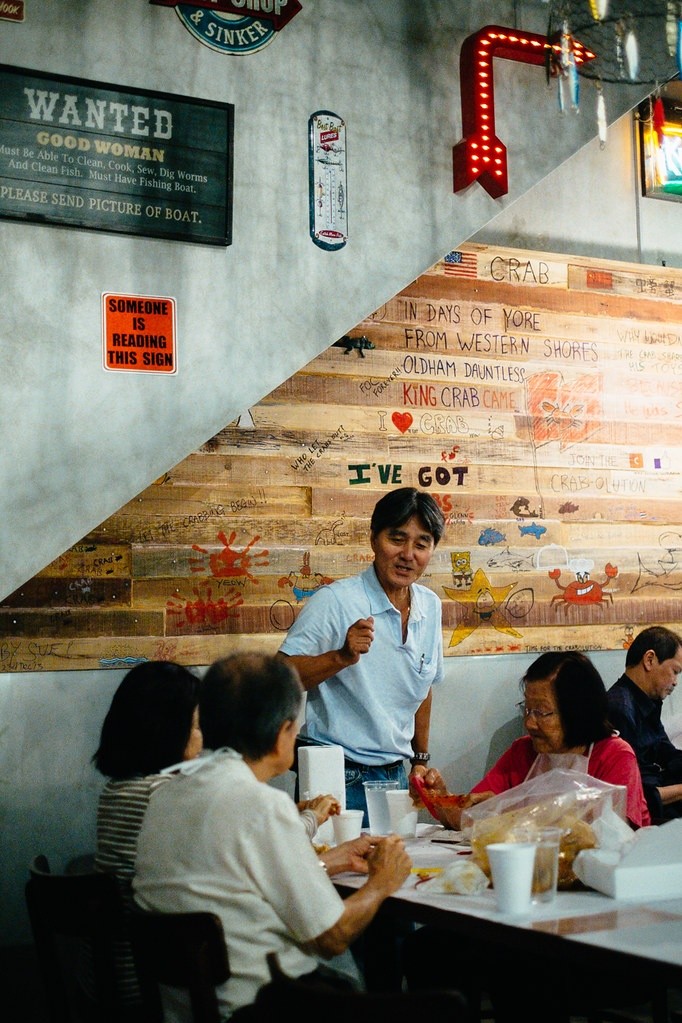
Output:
top-left (324, 821), bottom-right (682, 1023)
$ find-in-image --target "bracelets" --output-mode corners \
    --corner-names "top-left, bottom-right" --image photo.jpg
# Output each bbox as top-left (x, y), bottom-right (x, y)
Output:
top-left (414, 753), bottom-right (430, 761)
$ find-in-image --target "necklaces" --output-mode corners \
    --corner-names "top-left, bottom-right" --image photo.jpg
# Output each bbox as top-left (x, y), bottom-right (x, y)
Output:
top-left (401, 606), bottom-right (410, 624)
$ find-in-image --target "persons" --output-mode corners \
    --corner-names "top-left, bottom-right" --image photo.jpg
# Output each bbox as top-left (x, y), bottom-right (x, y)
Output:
top-left (274, 487), bottom-right (446, 827)
top-left (409, 650), bottom-right (650, 885)
top-left (91, 660), bottom-right (469, 1023)
top-left (606, 626), bottom-right (682, 826)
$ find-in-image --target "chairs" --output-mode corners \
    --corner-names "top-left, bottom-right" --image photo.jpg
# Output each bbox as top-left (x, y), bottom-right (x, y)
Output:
top-left (125, 900), bottom-right (233, 1023)
top-left (25, 854), bottom-right (125, 1023)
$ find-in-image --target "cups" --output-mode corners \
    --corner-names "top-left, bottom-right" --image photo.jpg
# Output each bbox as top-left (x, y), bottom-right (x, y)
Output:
top-left (484, 842), bottom-right (536, 912)
top-left (386, 790), bottom-right (418, 839)
top-left (512, 826), bottom-right (564, 904)
top-left (362, 780), bottom-right (399, 836)
top-left (331, 809), bottom-right (364, 847)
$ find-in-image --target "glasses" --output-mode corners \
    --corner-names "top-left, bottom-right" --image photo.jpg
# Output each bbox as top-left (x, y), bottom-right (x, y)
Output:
top-left (517, 700), bottom-right (558, 722)
